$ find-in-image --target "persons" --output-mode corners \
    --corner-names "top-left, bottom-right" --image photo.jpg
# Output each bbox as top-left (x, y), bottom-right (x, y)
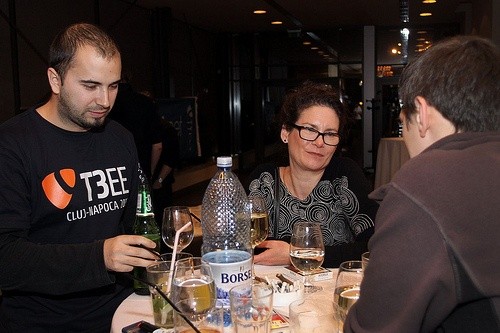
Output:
top-left (109, 86), bottom-right (182, 255)
top-left (0, 23), bottom-right (153, 333)
top-left (237, 81), bottom-right (380, 268)
top-left (343, 36), bottom-right (500, 333)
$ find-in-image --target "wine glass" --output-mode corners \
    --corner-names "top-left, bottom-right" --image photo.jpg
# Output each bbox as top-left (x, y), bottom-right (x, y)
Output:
top-left (289, 222), bottom-right (325, 295)
top-left (249, 197), bottom-right (269, 285)
top-left (162, 206), bottom-right (194, 260)
top-left (172, 257), bottom-right (216, 333)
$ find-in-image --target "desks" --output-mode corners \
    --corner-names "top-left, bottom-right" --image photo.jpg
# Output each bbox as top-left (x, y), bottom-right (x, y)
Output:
top-left (110, 263), bottom-right (366, 333)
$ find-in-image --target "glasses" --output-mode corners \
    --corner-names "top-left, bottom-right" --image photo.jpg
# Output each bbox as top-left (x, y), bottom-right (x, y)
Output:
top-left (285, 122), bottom-right (342, 146)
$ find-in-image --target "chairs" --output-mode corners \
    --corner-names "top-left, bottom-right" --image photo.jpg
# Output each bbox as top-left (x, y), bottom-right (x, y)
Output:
top-left (375, 138), bottom-right (410, 190)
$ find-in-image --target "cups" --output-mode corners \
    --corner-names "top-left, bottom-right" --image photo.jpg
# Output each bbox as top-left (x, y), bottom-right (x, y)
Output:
top-left (147, 261), bottom-right (185, 329)
top-left (156, 253), bottom-right (195, 279)
top-left (173, 297), bottom-right (225, 333)
top-left (338, 287), bottom-right (362, 333)
top-left (229, 284), bottom-right (273, 333)
top-left (288, 297), bottom-right (340, 333)
top-left (334, 261), bottom-right (364, 321)
top-left (362, 252), bottom-right (370, 271)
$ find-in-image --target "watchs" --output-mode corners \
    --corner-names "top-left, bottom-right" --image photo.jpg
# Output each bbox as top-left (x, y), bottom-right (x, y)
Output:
top-left (157, 177), bottom-right (165, 184)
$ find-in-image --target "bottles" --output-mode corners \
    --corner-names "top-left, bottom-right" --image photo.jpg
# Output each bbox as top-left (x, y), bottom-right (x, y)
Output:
top-left (134, 170), bottom-right (161, 296)
top-left (202, 156), bottom-right (253, 329)
